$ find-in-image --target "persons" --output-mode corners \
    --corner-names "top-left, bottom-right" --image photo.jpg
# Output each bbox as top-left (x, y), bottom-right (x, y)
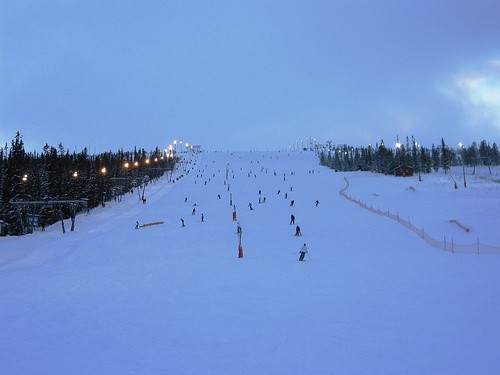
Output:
top-left (298, 243), bottom-right (309, 261)
top-left (295, 225), bottom-right (303, 236)
top-left (236, 222), bottom-right (242, 233)
top-left (104, 182), bottom-right (320, 228)
top-left (290, 213), bottom-right (295, 224)
